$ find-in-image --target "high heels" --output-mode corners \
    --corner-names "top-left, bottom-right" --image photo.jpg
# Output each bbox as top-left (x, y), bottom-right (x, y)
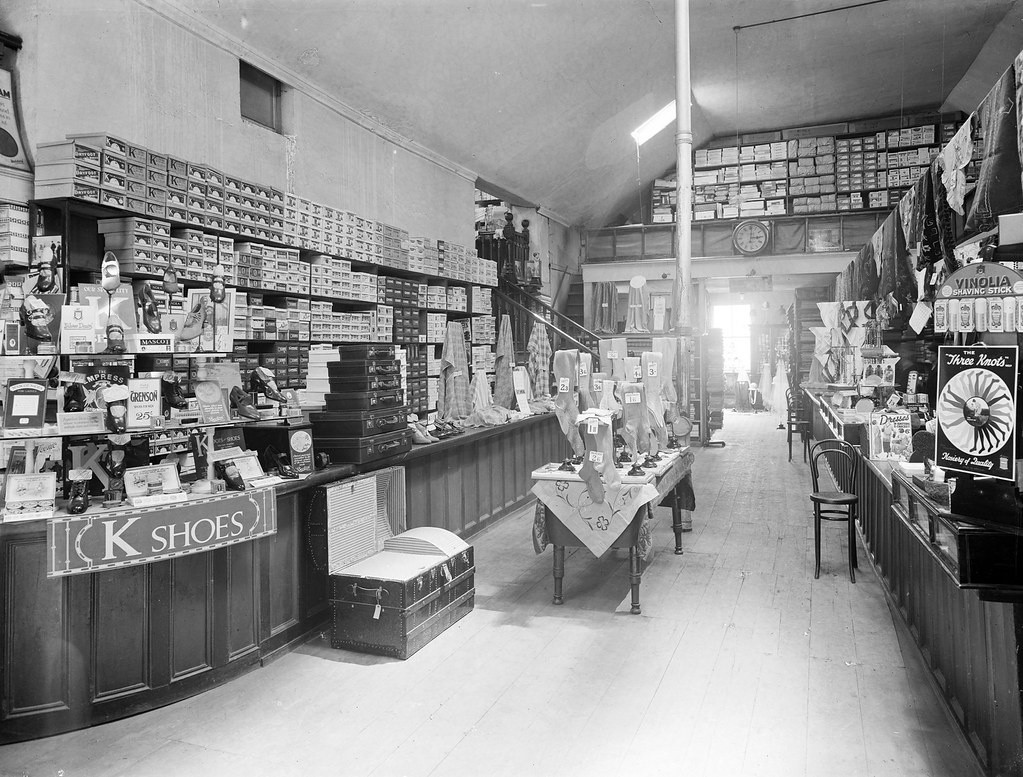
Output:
top-left (37, 255), bottom-right (58, 292)
top-left (250, 369), bottom-right (288, 403)
top-left (161, 376), bottom-right (189, 409)
top-left (264, 446), bottom-right (300, 479)
top-left (138, 283), bottom-right (162, 334)
top-left (19, 303), bottom-right (52, 342)
top-left (180, 303), bottom-right (207, 341)
top-left (229, 386), bottom-right (261, 421)
top-left (214, 460), bottom-right (245, 491)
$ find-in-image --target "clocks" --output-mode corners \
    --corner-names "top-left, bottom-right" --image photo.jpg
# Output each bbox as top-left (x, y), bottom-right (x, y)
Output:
top-left (731, 219), bottom-right (771, 256)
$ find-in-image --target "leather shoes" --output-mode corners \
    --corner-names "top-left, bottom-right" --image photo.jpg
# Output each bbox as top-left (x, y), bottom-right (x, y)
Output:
top-left (105, 440), bottom-right (130, 478)
top-left (66, 480), bottom-right (88, 514)
top-left (63, 383), bottom-right (85, 413)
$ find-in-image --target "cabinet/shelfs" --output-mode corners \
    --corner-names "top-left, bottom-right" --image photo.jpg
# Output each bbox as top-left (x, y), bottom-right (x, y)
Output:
top-left (648, 121), bottom-right (943, 223)
top-left (580, 333), bottom-right (724, 447)
top-left (28, 194), bottom-right (501, 450)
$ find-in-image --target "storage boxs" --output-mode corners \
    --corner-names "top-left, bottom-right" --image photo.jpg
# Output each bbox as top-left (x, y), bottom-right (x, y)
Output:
top-left (650, 109), bottom-right (981, 222)
top-left (0, 129), bottom-right (503, 522)
top-left (305, 467), bottom-right (478, 658)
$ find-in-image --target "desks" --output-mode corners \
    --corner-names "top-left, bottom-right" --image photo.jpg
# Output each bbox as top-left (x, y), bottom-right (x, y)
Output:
top-left (535, 448), bottom-right (694, 613)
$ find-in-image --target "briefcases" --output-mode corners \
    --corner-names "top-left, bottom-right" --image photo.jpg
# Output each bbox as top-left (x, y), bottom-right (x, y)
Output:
top-left (309, 345), bottom-right (412, 464)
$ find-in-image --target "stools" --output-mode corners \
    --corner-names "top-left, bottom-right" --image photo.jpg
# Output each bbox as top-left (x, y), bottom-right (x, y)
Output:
top-left (786, 388), bottom-right (812, 463)
top-left (808, 438), bottom-right (859, 584)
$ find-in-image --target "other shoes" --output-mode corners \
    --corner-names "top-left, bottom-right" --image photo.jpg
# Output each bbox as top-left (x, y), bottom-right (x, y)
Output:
top-left (104, 399), bottom-right (126, 435)
top-left (407, 411), bottom-right (467, 444)
top-left (106, 325), bottom-right (127, 352)
top-left (101, 250), bottom-right (120, 290)
top-left (514, 394), bottom-right (560, 415)
top-left (210, 274), bottom-right (226, 303)
top-left (163, 265), bottom-right (179, 293)
